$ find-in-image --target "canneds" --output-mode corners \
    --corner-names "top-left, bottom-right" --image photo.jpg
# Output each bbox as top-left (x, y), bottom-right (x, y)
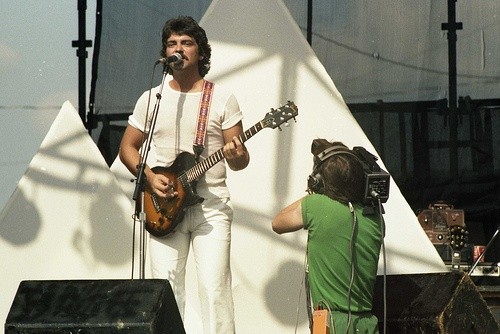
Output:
top-left (472, 245), bottom-right (486, 263)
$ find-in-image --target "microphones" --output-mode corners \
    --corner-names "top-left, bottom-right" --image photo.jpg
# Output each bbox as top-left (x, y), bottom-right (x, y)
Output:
top-left (158, 53), bottom-right (182, 65)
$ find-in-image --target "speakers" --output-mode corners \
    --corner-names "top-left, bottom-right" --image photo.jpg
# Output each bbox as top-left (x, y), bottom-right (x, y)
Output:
top-left (375, 272), bottom-right (500, 334)
top-left (4, 279), bottom-right (187, 334)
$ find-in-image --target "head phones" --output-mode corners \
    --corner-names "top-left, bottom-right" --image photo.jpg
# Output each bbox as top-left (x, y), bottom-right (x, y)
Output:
top-left (308, 145), bottom-right (359, 194)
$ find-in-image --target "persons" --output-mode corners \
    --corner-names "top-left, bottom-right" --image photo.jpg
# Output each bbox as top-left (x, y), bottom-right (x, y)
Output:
top-left (119, 16), bottom-right (250, 334)
top-left (272, 138), bottom-right (386, 334)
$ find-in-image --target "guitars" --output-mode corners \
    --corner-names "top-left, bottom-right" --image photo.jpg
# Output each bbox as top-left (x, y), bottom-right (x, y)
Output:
top-left (136, 101), bottom-right (298, 236)
